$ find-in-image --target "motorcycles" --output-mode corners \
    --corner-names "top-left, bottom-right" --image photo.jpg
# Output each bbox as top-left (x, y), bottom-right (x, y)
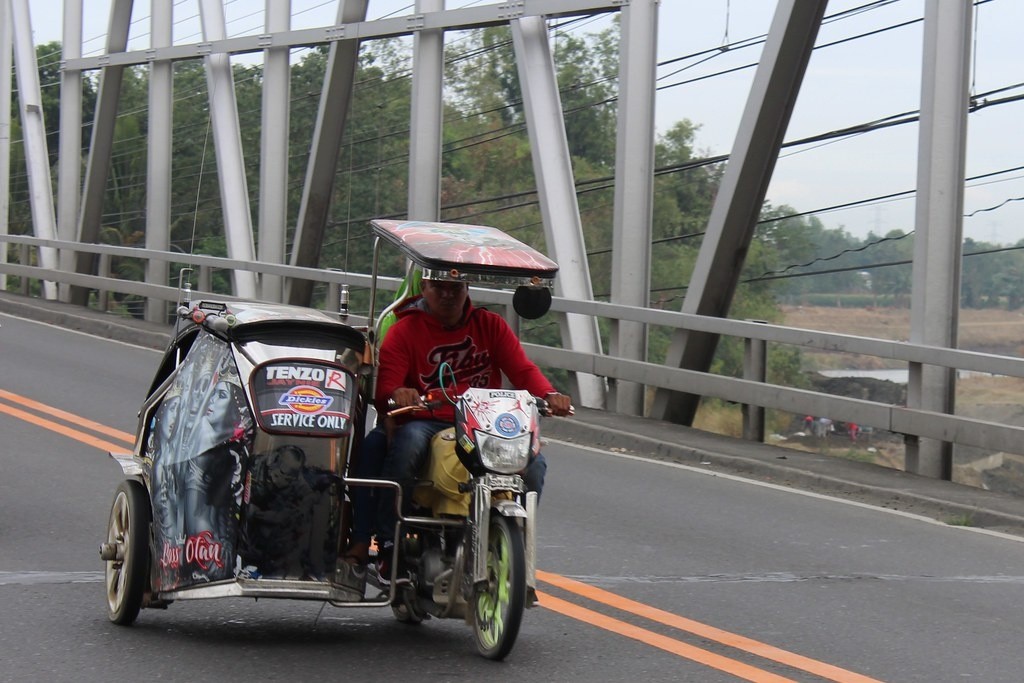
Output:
top-left (99, 218), bottom-right (578, 662)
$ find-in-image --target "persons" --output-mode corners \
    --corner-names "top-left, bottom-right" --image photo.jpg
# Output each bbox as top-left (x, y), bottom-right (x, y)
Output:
top-left (847, 422), bottom-right (858, 441)
top-left (336, 256), bottom-right (421, 572)
top-left (374, 279), bottom-right (570, 589)
top-left (802, 416), bottom-right (814, 434)
top-left (154, 382), bottom-right (246, 585)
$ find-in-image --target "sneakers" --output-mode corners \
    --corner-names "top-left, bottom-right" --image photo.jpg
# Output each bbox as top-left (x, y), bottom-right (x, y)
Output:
top-left (531, 591), bottom-right (541, 606)
top-left (375, 551), bottom-right (412, 585)
